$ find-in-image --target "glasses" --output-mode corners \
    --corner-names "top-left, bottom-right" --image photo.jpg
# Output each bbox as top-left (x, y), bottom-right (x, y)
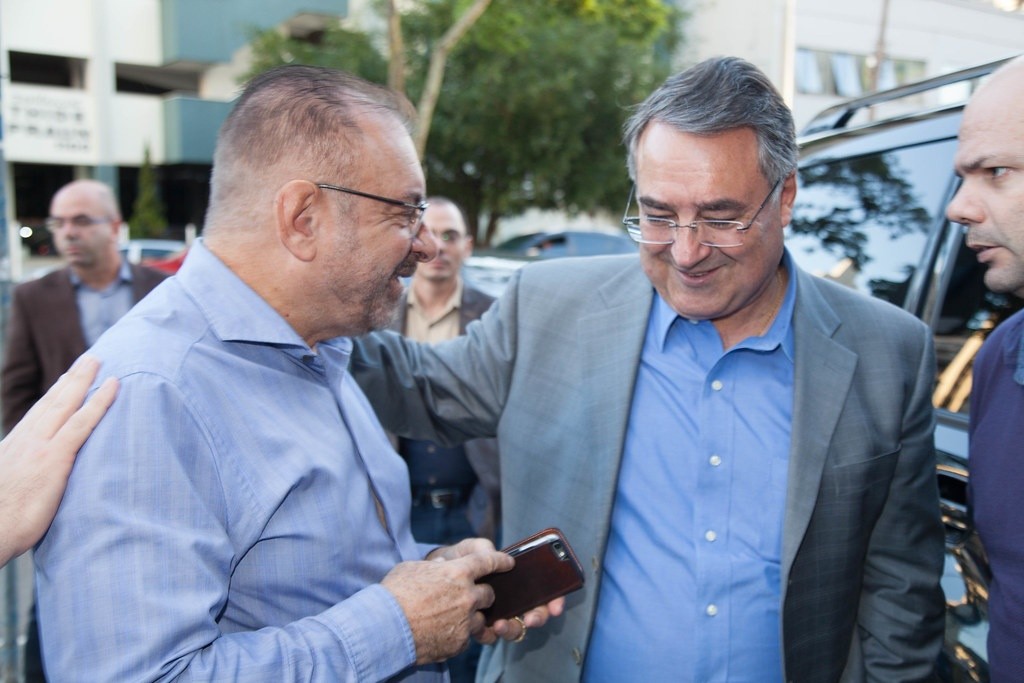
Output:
top-left (623, 182), bottom-right (780, 247)
top-left (43, 213), bottom-right (110, 233)
top-left (318, 183), bottom-right (427, 225)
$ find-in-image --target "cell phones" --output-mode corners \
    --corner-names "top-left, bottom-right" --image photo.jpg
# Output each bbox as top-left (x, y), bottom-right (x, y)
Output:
top-left (472, 527), bottom-right (583, 627)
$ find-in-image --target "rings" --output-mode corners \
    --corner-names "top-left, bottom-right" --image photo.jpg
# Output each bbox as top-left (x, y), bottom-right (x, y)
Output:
top-left (514, 616), bottom-right (527, 643)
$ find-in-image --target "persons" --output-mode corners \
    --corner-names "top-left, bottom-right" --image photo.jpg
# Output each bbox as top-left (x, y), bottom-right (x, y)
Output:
top-left (947, 49), bottom-right (1024, 683)
top-left (345, 55), bottom-right (946, 683)
top-left (34, 67), bottom-right (563, 683)
top-left (0, 177), bottom-right (502, 683)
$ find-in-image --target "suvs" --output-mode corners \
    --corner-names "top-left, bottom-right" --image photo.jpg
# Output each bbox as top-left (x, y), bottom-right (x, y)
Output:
top-left (783, 49), bottom-right (1024, 683)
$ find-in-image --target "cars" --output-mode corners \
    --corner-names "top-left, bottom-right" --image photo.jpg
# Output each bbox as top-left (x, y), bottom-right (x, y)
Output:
top-left (472, 229), bottom-right (641, 258)
top-left (119, 237), bottom-right (187, 275)
